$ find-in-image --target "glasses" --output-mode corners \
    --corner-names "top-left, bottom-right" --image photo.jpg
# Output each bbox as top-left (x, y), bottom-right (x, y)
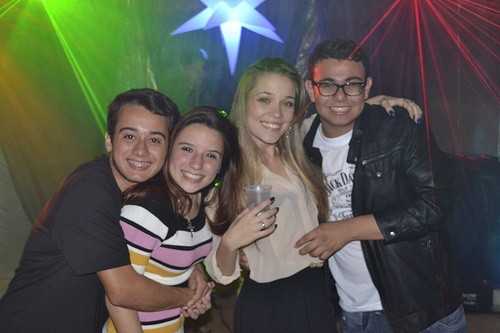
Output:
top-left (314, 81), bottom-right (367, 96)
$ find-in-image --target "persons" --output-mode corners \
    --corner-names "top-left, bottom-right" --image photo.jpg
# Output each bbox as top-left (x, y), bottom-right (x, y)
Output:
top-left (239, 39), bottom-right (466, 333)
top-left (102, 106), bottom-right (245, 333)
top-left (203, 57), bottom-right (424, 333)
top-left (0, 88), bottom-right (210, 333)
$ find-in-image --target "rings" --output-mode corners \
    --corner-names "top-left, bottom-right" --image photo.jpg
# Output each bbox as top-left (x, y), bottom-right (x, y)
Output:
top-left (261, 222), bottom-right (265, 230)
top-left (402, 101), bottom-right (408, 107)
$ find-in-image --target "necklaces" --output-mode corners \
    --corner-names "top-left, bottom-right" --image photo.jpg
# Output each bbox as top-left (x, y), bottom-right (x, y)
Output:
top-left (178, 193), bottom-right (195, 238)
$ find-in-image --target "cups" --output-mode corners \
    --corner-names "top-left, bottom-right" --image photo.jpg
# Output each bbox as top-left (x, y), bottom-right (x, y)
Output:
top-left (244, 185), bottom-right (272, 217)
